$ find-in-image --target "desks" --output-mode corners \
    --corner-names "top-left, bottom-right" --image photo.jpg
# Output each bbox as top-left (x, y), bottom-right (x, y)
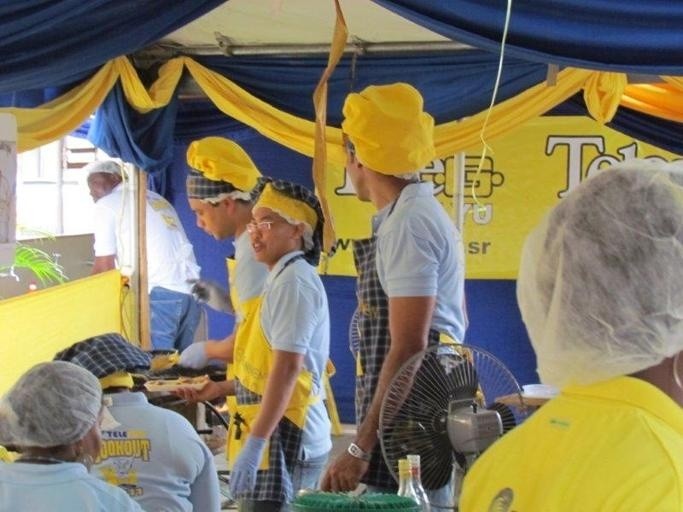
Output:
top-left (495, 393), bottom-right (550, 409)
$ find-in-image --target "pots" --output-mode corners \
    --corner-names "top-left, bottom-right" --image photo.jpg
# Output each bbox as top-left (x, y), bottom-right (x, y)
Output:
top-left (146, 348), bottom-right (181, 369)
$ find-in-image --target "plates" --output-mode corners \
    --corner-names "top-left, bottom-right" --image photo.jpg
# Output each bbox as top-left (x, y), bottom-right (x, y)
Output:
top-left (146, 379), bottom-right (209, 393)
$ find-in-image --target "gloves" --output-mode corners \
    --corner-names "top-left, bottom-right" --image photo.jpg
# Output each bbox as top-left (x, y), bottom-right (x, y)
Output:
top-left (228, 436), bottom-right (266, 492)
top-left (179, 341), bottom-right (208, 369)
top-left (186, 279), bottom-right (234, 315)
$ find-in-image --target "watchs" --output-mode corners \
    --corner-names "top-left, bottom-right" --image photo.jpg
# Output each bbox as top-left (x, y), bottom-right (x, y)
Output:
top-left (345, 441), bottom-right (371, 463)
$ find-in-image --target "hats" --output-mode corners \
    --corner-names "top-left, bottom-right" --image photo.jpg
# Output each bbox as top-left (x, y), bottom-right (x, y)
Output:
top-left (342, 82), bottom-right (436, 174)
top-left (186, 136), bottom-right (324, 231)
top-left (516, 159), bottom-right (683, 386)
top-left (0, 334), bottom-right (150, 447)
top-left (87, 160), bottom-right (128, 175)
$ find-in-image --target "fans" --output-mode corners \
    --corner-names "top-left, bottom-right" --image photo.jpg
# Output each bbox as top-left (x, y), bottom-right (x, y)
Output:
top-left (348, 303), bottom-right (361, 361)
top-left (377, 339), bottom-right (529, 506)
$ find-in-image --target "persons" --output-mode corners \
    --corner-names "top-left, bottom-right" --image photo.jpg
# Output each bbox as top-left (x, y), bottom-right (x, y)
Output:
top-left (308, 80), bottom-right (470, 512)
top-left (0, 358), bottom-right (146, 511)
top-left (457, 158), bottom-right (683, 512)
top-left (84, 156), bottom-right (202, 354)
top-left (52, 330), bottom-right (222, 512)
top-left (165, 136), bottom-right (269, 421)
top-left (164, 176), bottom-right (335, 512)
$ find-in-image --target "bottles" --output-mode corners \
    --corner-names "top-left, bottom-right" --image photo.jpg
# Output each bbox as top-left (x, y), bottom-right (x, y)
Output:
top-left (406, 454), bottom-right (431, 511)
top-left (395, 458), bottom-right (419, 504)
